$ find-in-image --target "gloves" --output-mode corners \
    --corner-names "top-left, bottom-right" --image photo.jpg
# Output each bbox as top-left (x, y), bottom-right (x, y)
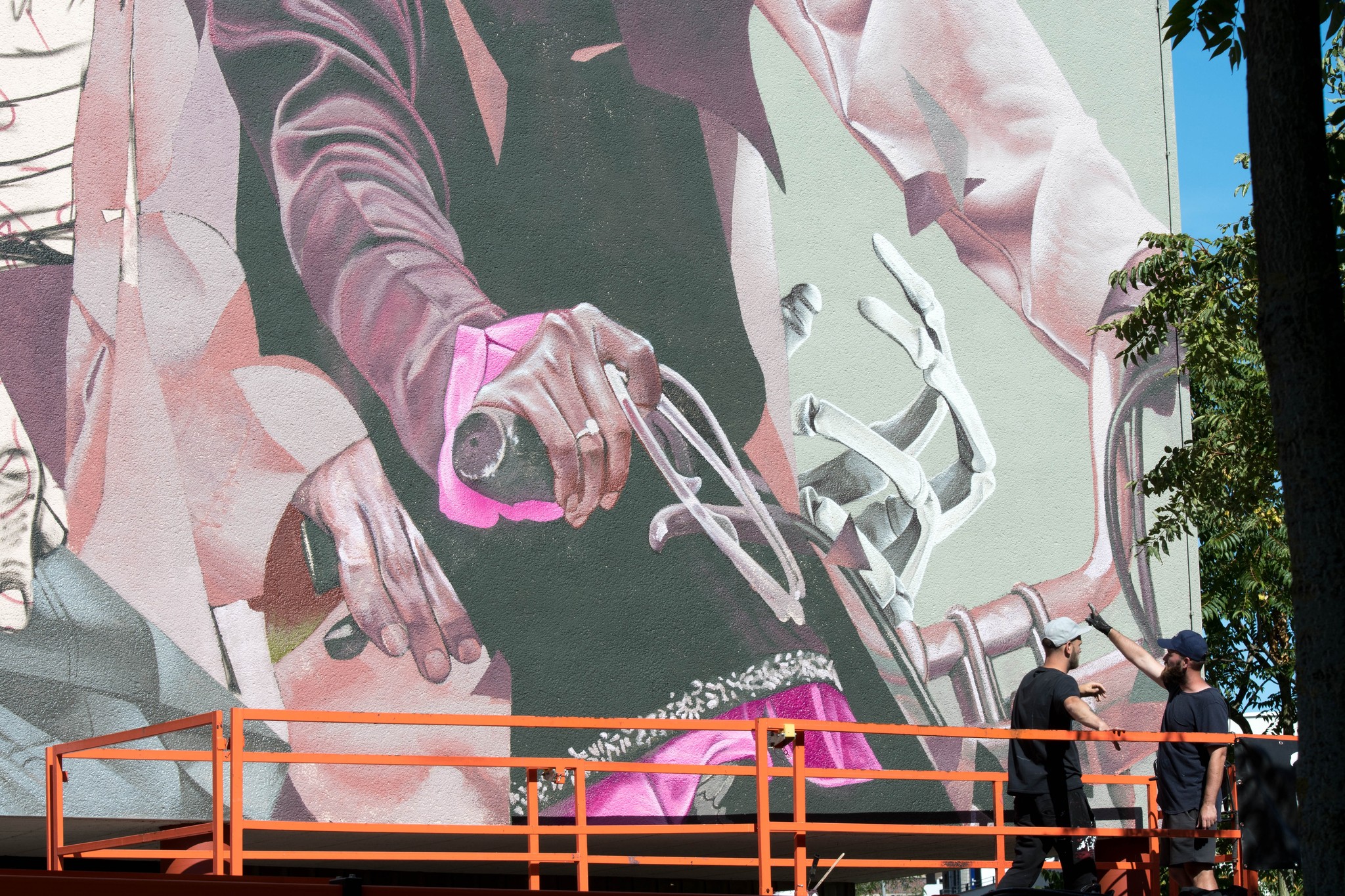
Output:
top-left (1085, 602), bottom-right (1113, 637)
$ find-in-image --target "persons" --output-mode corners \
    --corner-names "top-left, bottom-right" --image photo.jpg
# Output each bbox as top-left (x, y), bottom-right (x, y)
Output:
top-left (1084, 601), bottom-right (1229, 896)
top-left (995, 617), bottom-right (1126, 891)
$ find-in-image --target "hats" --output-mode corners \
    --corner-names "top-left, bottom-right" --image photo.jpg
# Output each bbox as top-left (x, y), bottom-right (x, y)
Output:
top-left (1157, 630), bottom-right (1207, 662)
top-left (1040, 617), bottom-right (1092, 647)
